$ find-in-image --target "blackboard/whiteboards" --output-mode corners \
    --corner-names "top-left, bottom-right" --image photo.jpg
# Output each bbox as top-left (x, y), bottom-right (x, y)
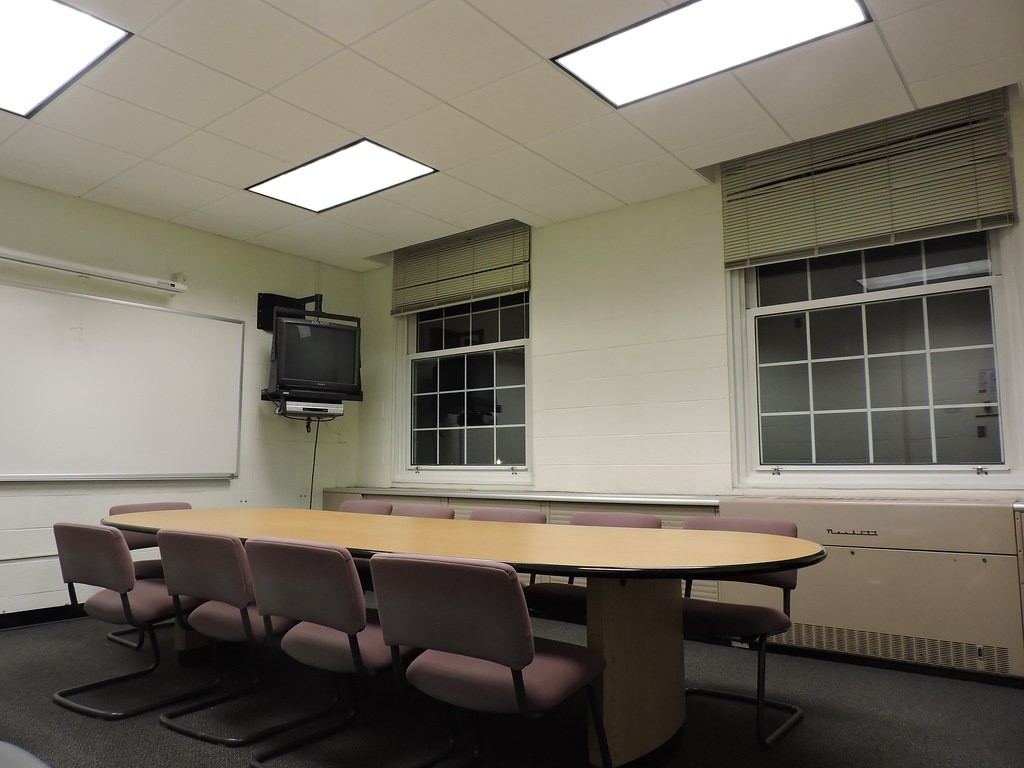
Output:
top-left (0, 279), bottom-right (245, 482)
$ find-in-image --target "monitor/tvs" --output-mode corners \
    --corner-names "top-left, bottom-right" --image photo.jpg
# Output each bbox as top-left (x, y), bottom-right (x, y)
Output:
top-left (268, 317), bottom-right (362, 394)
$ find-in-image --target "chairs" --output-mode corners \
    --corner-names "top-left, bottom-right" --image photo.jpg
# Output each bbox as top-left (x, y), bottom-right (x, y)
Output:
top-left (53, 522), bottom-right (221, 721)
top-left (368, 551), bottom-right (611, 768)
top-left (154, 527), bottom-right (339, 747)
top-left (394, 504), bottom-right (456, 519)
top-left (339, 500), bottom-right (393, 514)
top-left (679, 515), bottom-right (804, 748)
top-left (468, 506), bottom-right (548, 588)
top-left (242, 534), bottom-right (454, 768)
top-left (106, 503), bottom-right (192, 650)
top-left (518, 513), bottom-right (660, 623)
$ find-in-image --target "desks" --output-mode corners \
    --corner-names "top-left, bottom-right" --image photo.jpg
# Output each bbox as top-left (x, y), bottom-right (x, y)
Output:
top-left (101, 507), bottom-right (829, 768)
